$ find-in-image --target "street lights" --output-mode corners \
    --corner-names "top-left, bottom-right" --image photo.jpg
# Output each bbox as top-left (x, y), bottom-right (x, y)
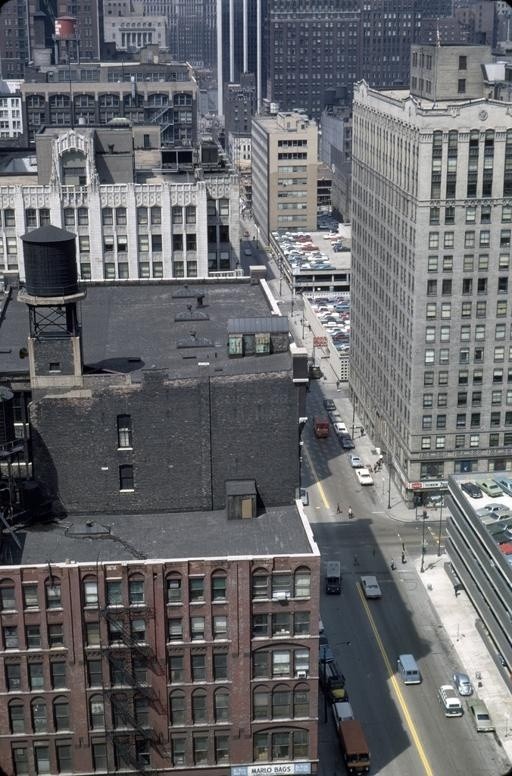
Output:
top-left (377, 448), bottom-right (394, 510)
top-left (417, 507), bottom-right (431, 575)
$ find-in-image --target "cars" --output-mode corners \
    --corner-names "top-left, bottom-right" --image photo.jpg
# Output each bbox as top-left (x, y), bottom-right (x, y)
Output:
top-left (244, 247), bottom-right (253, 257)
top-left (242, 230), bottom-right (251, 238)
top-left (452, 671), bottom-right (475, 697)
top-left (346, 453), bottom-right (363, 467)
top-left (323, 397), bottom-right (356, 450)
top-left (359, 574), bottom-right (383, 600)
top-left (465, 697), bottom-right (496, 733)
top-left (458, 476), bottom-right (512, 568)
top-left (306, 296), bottom-right (352, 356)
top-left (271, 207), bottom-right (351, 273)
top-left (314, 608), bottom-right (357, 733)
top-left (354, 467), bottom-right (376, 485)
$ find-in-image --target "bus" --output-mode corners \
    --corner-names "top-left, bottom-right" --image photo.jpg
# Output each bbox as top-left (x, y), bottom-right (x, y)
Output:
top-left (436, 684), bottom-right (464, 719)
top-left (335, 719), bottom-right (374, 775)
top-left (326, 560), bottom-right (343, 596)
top-left (396, 652), bottom-right (422, 687)
top-left (313, 414), bottom-right (331, 440)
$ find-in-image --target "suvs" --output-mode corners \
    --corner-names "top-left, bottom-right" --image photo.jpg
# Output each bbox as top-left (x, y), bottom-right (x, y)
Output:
top-left (307, 364), bottom-right (323, 381)
